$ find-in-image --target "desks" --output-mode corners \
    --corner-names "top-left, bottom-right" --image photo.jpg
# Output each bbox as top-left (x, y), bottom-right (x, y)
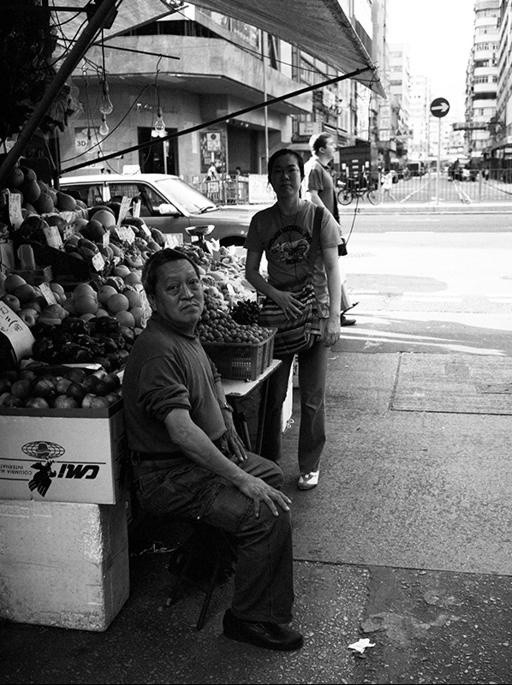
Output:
top-left (118, 358), bottom-right (282, 456)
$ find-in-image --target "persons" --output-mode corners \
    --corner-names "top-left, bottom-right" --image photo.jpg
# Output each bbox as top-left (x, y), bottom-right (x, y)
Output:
top-left (301, 132), bottom-right (356, 325)
top-left (379, 169), bottom-right (397, 204)
top-left (244, 149), bottom-right (344, 490)
top-left (122, 249), bottom-right (303, 651)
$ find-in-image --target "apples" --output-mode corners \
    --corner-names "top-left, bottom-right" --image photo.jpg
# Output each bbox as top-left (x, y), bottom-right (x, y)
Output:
top-left (3, 274), bottom-right (146, 337)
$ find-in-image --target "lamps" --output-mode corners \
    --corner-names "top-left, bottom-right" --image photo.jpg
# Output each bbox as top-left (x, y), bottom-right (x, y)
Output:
top-left (99, 114), bottom-right (109, 136)
top-left (99, 81), bottom-right (113, 115)
top-left (155, 110), bottom-right (165, 132)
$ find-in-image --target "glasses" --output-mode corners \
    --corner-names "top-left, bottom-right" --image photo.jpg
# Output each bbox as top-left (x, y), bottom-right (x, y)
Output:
top-left (272, 165), bottom-right (300, 176)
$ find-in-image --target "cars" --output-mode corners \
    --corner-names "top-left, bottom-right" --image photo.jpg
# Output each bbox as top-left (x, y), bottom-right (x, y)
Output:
top-left (55, 170), bottom-right (272, 250)
top-left (382, 157), bottom-right (479, 182)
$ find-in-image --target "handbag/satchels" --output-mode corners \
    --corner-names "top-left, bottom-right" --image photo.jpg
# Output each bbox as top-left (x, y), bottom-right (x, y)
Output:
top-left (259, 285), bottom-right (321, 359)
top-left (338, 238), bottom-right (347, 255)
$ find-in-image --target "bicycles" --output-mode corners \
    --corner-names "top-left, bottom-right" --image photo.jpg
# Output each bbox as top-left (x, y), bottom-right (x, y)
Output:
top-left (338, 173), bottom-right (378, 206)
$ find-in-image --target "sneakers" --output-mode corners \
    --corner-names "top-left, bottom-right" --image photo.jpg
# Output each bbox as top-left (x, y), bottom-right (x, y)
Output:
top-left (298, 468), bottom-right (320, 490)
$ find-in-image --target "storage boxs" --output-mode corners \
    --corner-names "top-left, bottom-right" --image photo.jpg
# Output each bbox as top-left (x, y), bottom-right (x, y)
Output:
top-left (1, 398), bottom-right (123, 505)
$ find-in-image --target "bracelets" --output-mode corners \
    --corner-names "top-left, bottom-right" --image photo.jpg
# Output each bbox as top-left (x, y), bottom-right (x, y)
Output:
top-left (220, 402), bottom-right (234, 412)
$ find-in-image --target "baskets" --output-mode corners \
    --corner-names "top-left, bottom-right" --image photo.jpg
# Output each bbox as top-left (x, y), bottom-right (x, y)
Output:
top-left (201, 327), bottom-right (278, 381)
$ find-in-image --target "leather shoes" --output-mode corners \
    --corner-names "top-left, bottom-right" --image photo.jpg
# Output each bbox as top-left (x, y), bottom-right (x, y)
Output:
top-left (341, 315), bottom-right (356, 326)
top-left (221, 610), bottom-right (303, 651)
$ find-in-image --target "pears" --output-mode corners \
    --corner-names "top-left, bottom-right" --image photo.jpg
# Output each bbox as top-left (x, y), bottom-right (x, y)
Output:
top-left (8, 157), bottom-right (77, 216)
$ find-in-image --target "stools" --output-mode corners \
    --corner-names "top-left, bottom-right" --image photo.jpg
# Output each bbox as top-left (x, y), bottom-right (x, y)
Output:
top-left (162, 514), bottom-right (236, 630)
